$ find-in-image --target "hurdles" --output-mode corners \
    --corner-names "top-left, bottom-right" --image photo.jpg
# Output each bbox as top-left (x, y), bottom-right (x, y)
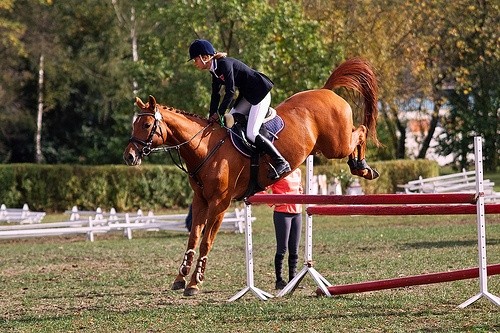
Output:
top-left (277, 154), bottom-right (500, 298)
top-left (227, 136), bottom-right (500, 309)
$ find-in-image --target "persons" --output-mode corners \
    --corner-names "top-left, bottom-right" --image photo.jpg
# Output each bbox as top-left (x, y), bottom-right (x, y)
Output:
top-left (188, 39), bottom-right (292, 181)
top-left (257, 168), bottom-right (306, 290)
top-left (185, 204), bottom-right (207, 235)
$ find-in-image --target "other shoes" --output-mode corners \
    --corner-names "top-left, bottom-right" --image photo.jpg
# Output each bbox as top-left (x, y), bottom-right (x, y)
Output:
top-left (297, 285), bottom-right (306, 289)
top-left (276, 281), bottom-right (287, 289)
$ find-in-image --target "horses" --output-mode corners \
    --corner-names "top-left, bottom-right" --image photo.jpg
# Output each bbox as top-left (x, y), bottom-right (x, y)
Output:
top-left (122, 57), bottom-right (385, 296)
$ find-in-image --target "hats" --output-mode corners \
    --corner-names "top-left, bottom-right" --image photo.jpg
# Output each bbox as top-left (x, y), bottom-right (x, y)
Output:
top-left (187, 39), bottom-right (215, 62)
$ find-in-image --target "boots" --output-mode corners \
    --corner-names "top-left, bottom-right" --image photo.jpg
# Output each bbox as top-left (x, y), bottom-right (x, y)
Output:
top-left (255, 134), bottom-right (291, 178)
